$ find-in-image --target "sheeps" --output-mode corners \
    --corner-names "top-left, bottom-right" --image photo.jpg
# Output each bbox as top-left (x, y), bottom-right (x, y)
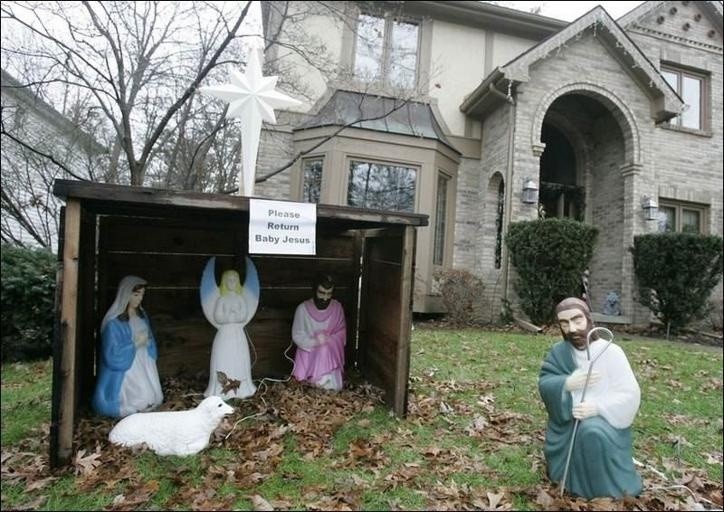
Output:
top-left (108, 396), bottom-right (235, 456)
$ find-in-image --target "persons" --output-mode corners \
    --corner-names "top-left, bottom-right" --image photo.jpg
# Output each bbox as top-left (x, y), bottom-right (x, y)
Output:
top-left (539, 297), bottom-right (643, 499)
top-left (90, 274), bottom-right (164, 419)
top-left (291, 272), bottom-right (347, 391)
top-left (199, 256), bottom-right (260, 400)
